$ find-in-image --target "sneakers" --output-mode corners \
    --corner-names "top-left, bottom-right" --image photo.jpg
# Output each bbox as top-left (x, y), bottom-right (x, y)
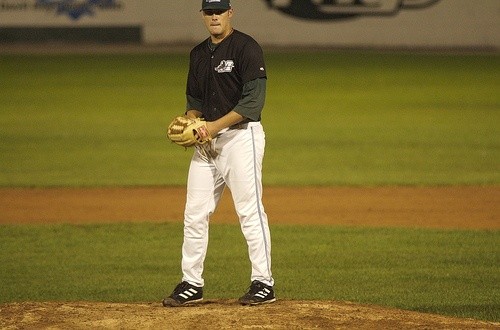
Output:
top-left (239, 280), bottom-right (276, 306)
top-left (161, 281), bottom-right (203, 306)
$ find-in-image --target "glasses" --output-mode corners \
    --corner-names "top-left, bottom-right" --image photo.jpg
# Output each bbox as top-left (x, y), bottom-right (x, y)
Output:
top-left (202, 10), bottom-right (227, 15)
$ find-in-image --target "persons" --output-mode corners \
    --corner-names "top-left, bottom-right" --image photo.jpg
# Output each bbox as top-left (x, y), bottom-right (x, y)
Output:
top-left (163, 0), bottom-right (276, 308)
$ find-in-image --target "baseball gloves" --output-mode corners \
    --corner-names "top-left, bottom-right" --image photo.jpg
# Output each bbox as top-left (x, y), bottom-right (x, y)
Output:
top-left (167, 115), bottom-right (212, 148)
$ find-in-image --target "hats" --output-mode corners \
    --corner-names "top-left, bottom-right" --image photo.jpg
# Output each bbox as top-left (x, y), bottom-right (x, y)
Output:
top-left (200, 0), bottom-right (231, 12)
